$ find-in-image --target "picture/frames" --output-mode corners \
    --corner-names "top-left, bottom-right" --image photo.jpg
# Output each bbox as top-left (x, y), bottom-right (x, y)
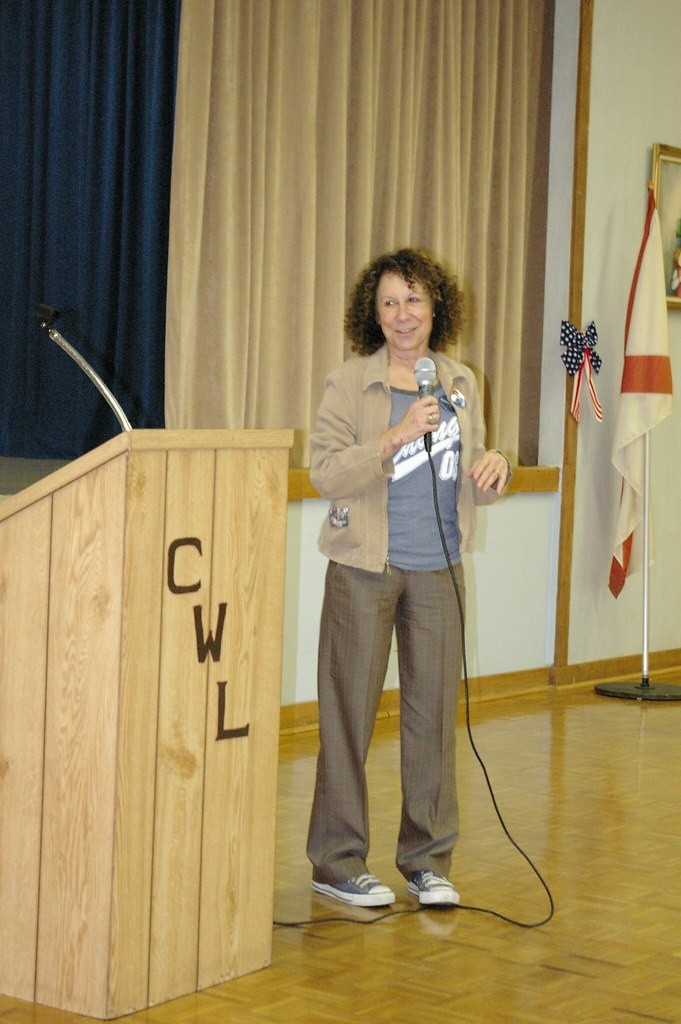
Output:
top-left (651, 143), bottom-right (681, 309)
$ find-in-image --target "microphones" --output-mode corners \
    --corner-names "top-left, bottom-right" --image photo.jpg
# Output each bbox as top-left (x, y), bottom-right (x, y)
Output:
top-left (413, 357), bottom-right (436, 452)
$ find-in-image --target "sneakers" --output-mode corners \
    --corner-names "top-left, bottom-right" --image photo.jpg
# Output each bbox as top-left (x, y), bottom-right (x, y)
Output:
top-left (407, 871), bottom-right (460, 906)
top-left (311, 873), bottom-right (396, 907)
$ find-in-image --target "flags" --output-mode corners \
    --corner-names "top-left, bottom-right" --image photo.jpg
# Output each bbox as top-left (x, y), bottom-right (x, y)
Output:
top-left (608, 190), bottom-right (675, 600)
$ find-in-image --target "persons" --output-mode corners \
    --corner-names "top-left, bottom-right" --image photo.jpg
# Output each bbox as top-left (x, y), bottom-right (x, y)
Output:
top-left (306, 247), bottom-right (511, 907)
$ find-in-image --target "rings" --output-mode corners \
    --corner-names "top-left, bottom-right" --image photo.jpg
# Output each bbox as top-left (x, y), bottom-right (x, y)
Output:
top-left (427, 414), bottom-right (436, 424)
top-left (493, 469), bottom-right (500, 477)
